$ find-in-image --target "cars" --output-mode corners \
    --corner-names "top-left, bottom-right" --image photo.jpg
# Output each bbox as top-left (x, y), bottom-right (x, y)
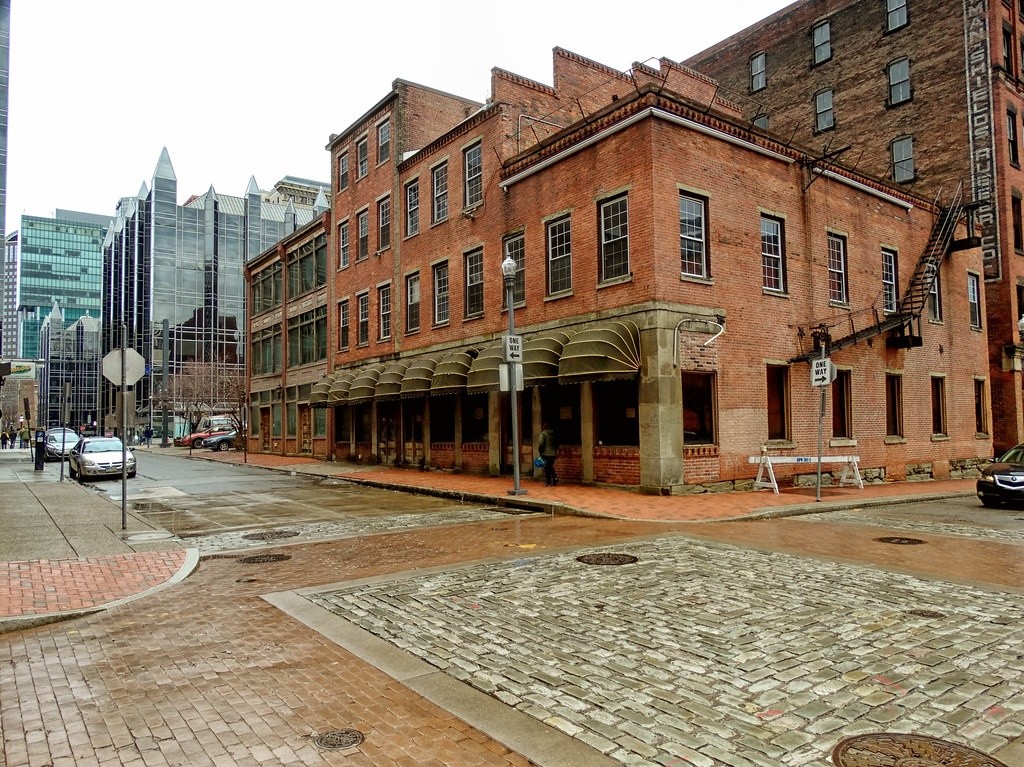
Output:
top-left (200, 427), bottom-right (246, 452)
top-left (181, 416), bottom-right (239, 449)
top-left (68, 436), bottom-right (137, 482)
top-left (44, 427), bottom-right (76, 444)
top-left (975, 441), bottom-right (1024, 507)
top-left (44, 432), bottom-right (82, 461)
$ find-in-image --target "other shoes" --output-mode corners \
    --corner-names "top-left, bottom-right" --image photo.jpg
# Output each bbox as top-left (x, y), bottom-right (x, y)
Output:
top-left (553, 477), bottom-right (558, 485)
top-left (544, 483), bottom-right (551, 486)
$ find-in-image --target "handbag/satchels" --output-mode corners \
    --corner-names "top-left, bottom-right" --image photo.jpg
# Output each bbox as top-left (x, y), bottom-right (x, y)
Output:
top-left (535, 456), bottom-right (545, 467)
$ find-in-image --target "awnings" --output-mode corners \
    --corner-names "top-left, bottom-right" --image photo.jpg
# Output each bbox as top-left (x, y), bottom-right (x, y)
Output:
top-left (306, 318), bottom-right (642, 407)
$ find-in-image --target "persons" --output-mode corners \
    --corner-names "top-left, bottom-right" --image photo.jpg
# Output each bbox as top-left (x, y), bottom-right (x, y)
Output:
top-left (136, 425), bottom-right (154, 448)
top-left (0, 425), bottom-right (32, 449)
top-left (537, 421), bottom-right (558, 486)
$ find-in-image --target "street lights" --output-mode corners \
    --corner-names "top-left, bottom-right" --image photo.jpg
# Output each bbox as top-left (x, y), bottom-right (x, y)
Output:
top-left (501, 251), bottom-right (528, 496)
top-left (20, 415), bottom-right (25, 448)
